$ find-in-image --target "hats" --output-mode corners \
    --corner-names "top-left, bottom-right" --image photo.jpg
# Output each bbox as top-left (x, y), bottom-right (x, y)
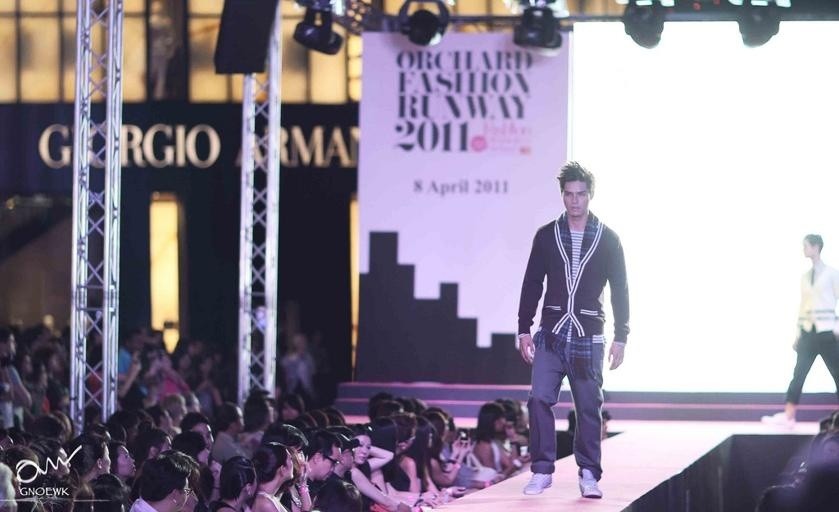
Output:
top-left (337, 433), bottom-right (360, 452)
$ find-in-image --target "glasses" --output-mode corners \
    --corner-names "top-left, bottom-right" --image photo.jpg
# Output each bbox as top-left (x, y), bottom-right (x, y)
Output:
top-left (324, 455), bottom-right (340, 466)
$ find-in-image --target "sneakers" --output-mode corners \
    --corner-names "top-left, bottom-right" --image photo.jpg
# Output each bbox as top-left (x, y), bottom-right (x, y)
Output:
top-left (578, 470), bottom-right (603, 498)
top-left (762, 413), bottom-right (795, 427)
top-left (524, 471), bottom-right (553, 494)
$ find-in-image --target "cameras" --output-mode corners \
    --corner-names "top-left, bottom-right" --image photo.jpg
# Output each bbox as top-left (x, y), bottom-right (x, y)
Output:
top-left (459, 428), bottom-right (469, 445)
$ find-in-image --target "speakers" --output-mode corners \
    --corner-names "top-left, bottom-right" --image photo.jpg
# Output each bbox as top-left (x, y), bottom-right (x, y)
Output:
top-left (212, 0), bottom-right (281, 76)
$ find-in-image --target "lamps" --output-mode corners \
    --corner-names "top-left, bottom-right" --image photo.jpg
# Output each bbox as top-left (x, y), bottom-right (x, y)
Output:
top-left (292, 0), bottom-right (787, 58)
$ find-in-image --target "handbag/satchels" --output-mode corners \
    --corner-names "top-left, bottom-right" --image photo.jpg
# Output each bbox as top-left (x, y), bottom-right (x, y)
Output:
top-left (458, 464), bottom-right (490, 487)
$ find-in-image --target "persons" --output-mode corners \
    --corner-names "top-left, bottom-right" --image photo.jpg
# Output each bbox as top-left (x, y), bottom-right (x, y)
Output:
top-left (754, 410), bottom-right (839, 511)
top-left (760, 233), bottom-right (838, 431)
top-left (566, 409), bottom-right (612, 456)
top-left (517, 161), bottom-right (630, 499)
